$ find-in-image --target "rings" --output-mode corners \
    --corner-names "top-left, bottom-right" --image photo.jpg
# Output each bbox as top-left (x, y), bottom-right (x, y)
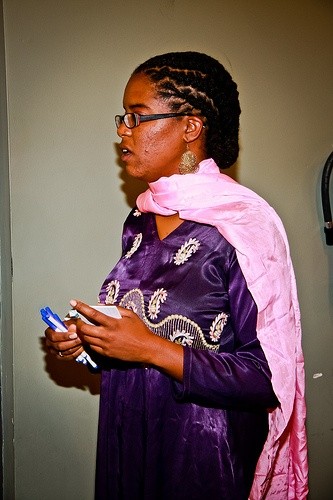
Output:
top-left (58, 352), bottom-right (64, 357)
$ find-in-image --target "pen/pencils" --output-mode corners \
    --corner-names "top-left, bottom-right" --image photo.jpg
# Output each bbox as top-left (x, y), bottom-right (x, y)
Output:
top-left (40, 305), bottom-right (98, 370)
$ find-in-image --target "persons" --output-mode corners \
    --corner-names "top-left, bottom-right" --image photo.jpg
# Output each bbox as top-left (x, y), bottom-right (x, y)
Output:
top-left (44, 50), bottom-right (309, 500)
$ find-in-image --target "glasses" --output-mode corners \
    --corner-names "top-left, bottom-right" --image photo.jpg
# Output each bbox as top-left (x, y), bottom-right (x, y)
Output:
top-left (114, 112), bottom-right (205, 129)
